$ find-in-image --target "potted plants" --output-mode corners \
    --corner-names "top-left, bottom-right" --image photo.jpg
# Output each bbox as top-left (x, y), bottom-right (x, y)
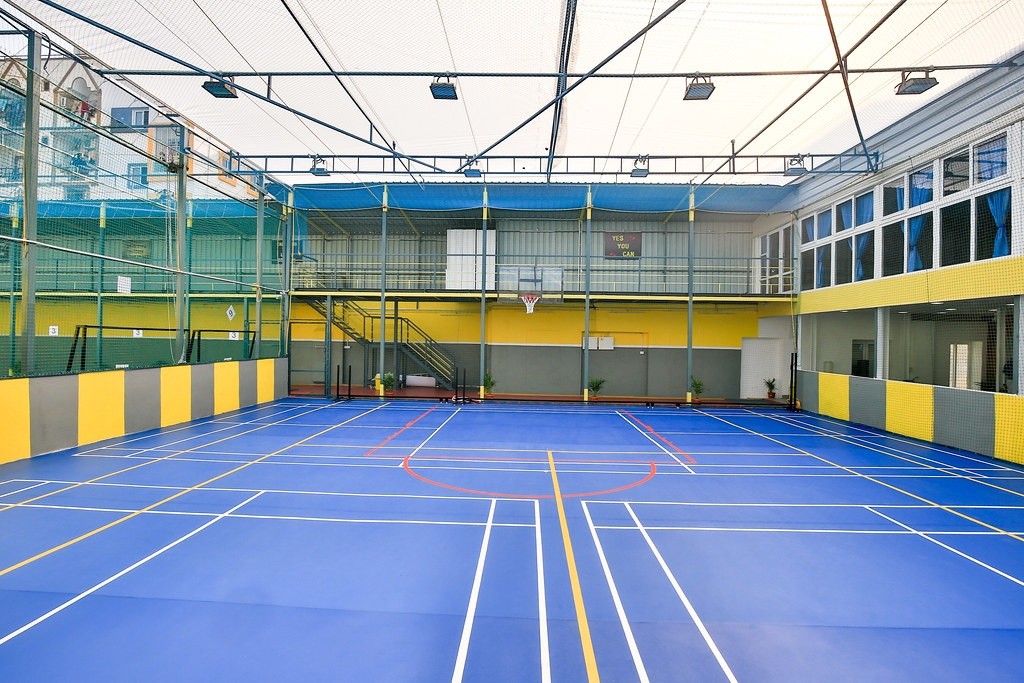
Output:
top-left (384, 373), bottom-right (397, 394)
top-left (483, 374), bottom-right (494, 395)
top-left (763, 376), bottom-right (777, 400)
top-left (691, 375), bottom-right (705, 402)
top-left (587, 379), bottom-right (605, 401)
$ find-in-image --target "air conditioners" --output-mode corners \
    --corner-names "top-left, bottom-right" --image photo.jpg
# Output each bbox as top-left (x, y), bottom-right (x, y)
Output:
top-left (61, 96), bottom-right (68, 106)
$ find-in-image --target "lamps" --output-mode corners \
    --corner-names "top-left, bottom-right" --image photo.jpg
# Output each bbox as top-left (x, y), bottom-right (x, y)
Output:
top-left (431, 70), bottom-right (460, 100)
top-left (682, 75), bottom-right (716, 100)
top-left (311, 154), bottom-right (331, 176)
top-left (897, 68), bottom-right (938, 97)
top-left (787, 153), bottom-right (807, 179)
top-left (631, 153), bottom-right (652, 177)
top-left (202, 73), bottom-right (236, 98)
top-left (462, 155), bottom-right (484, 176)
